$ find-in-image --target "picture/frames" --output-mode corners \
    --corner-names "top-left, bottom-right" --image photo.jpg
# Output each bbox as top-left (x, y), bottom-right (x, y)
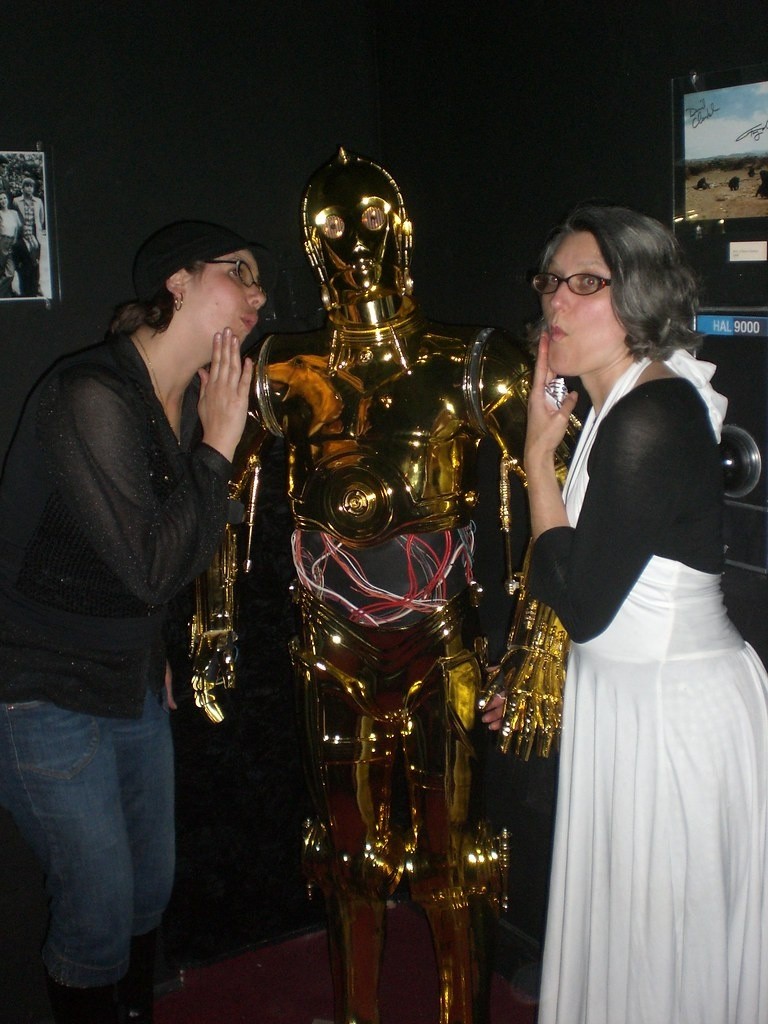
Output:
top-left (1, 141), bottom-right (62, 305)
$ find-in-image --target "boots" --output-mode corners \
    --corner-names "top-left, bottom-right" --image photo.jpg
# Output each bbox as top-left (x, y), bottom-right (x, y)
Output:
top-left (111, 928), bottom-right (158, 1024)
top-left (43, 965), bottom-right (114, 1024)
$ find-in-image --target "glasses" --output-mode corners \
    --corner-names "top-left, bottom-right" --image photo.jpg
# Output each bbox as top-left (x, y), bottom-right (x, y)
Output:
top-left (531, 273), bottom-right (615, 295)
top-left (204, 259), bottom-right (265, 296)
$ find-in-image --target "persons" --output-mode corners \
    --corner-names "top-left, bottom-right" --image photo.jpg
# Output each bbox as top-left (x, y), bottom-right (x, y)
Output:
top-left (523, 205), bottom-right (768, 1024)
top-left (0, 216), bottom-right (271, 1024)
top-left (0, 180), bottom-right (45, 298)
top-left (189, 146), bottom-right (583, 1024)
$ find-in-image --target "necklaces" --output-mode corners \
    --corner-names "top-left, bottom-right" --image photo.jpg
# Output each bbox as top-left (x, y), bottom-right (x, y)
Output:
top-left (132, 328), bottom-right (164, 410)
